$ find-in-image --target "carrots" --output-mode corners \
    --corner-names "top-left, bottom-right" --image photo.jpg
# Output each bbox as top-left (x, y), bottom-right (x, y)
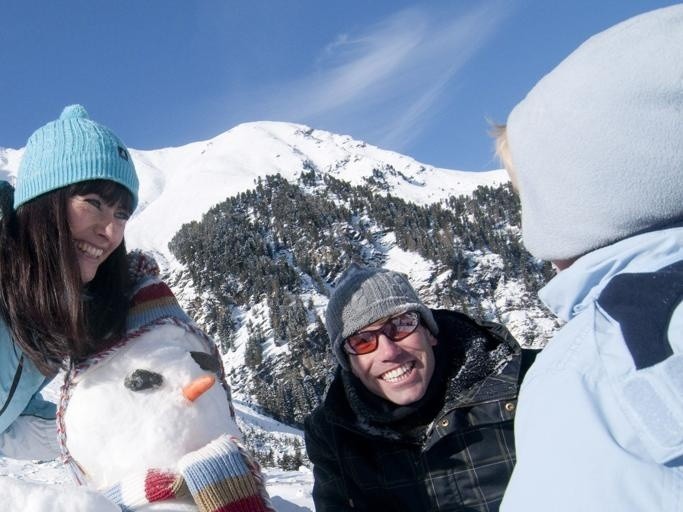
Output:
top-left (183, 375), bottom-right (216, 401)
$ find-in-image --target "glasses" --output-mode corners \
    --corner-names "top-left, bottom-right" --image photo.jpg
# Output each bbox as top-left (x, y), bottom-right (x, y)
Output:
top-left (338, 311), bottom-right (419, 356)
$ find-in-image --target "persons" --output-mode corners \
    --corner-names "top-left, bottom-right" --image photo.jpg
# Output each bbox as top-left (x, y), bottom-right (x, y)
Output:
top-left (305, 272), bottom-right (537, 512)
top-left (490, 0), bottom-right (682, 512)
top-left (1, 104), bottom-right (136, 455)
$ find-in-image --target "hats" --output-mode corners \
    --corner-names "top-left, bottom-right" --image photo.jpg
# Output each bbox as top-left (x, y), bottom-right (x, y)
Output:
top-left (9, 102), bottom-right (139, 212)
top-left (53, 248), bottom-right (217, 382)
top-left (505, 3), bottom-right (682, 260)
top-left (321, 261), bottom-right (441, 373)
